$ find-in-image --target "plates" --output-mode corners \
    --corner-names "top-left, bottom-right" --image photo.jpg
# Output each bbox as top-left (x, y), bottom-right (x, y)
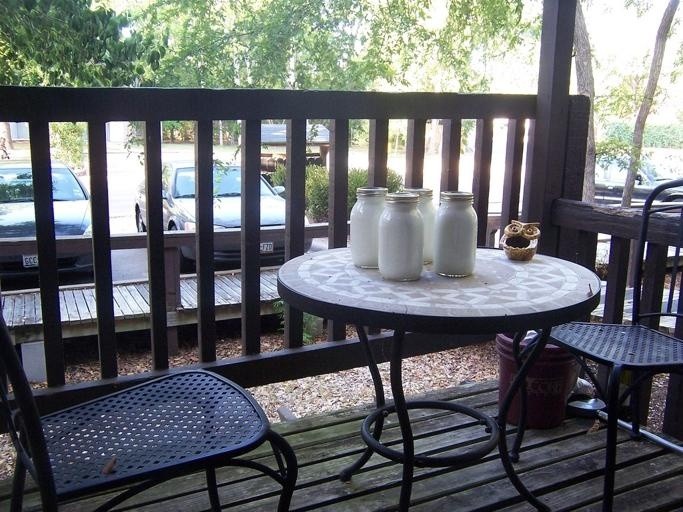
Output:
top-left (568, 394), bottom-right (606, 418)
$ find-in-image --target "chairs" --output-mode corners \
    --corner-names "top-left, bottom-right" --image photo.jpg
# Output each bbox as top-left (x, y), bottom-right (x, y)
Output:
top-left (504, 171), bottom-right (682, 511)
top-left (0, 295), bottom-right (299, 511)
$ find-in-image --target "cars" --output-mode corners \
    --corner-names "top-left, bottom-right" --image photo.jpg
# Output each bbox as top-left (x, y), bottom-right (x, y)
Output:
top-left (0, 159), bottom-right (93, 284)
top-left (134, 158), bottom-right (315, 271)
top-left (593, 144), bottom-right (683, 204)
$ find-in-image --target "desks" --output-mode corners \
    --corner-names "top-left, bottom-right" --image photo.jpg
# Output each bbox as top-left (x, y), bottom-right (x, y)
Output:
top-left (274, 240), bottom-right (605, 510)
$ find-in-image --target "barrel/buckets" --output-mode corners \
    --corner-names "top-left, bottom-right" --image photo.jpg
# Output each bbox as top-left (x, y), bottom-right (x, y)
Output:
top-left (495, 329), bottom-right (575, 429)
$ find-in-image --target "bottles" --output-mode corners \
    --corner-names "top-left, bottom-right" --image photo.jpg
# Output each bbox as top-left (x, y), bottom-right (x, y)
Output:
top-left (431, 190), bottom-right (476, 279)
top-left (348, 187), bottom-right (390, 270)
top-left (405, 188), bottom-right (436, 268)
top-left (377, 193), bottom-right (425, 283)
